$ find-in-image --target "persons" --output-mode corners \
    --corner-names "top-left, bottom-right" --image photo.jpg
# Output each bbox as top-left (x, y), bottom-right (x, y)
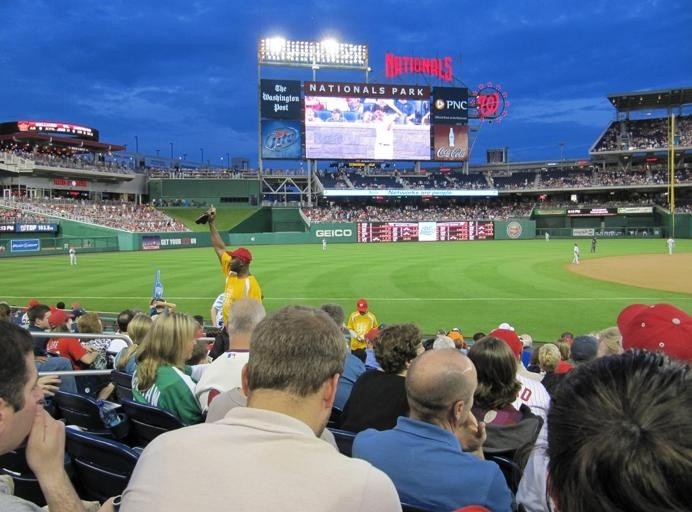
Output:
top-left (304, 94), bottom-right (431, 128)
top-left (1, 292), bottom-right (691, 511)
top-left (207, 205), bottom-right (262, 335)
top-left (1, 116), bottom-right (692, 265)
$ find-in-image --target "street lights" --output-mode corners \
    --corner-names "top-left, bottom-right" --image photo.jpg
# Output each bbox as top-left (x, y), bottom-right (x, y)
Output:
top-left (131, 134), bottom-right (231, 170)
top-left (502, 145), bottom-right (510, 163)
top-left (558, 142), bottom-right (564, 161)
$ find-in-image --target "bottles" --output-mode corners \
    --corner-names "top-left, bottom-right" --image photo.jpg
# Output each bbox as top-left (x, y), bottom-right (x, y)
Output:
top-left (96, 398), bottom-right (122, 428)
